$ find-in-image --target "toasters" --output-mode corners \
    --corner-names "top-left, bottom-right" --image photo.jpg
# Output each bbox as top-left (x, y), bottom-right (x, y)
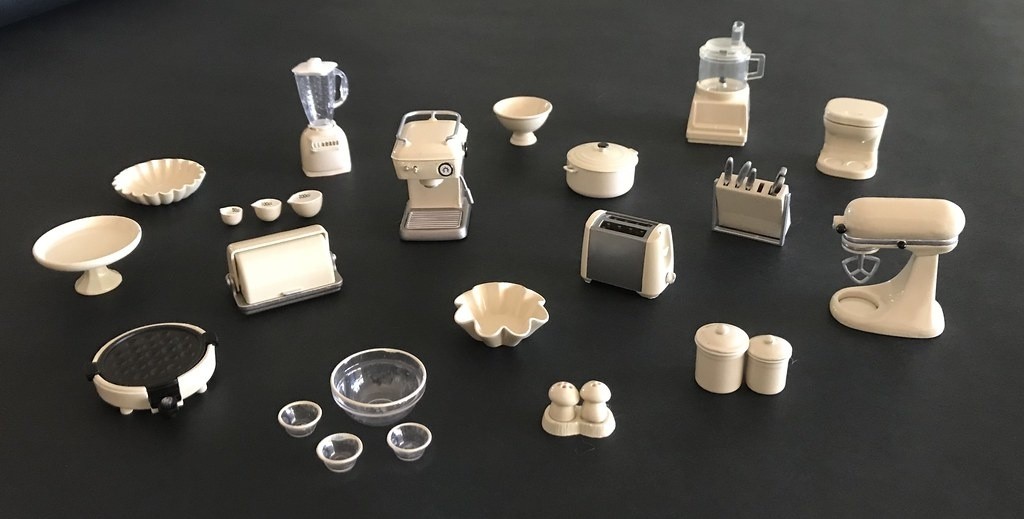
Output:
top-left (581, 210), bottom-right (678, 299)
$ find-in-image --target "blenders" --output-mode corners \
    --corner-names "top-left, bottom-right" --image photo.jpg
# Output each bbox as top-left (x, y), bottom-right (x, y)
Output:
top-left (393, 108), bottom-right (474, 243)
top-left (292, 57), bottom-right (352, 178)
top-left (831, 198), bottom-right (967, 342)
top-left (686, 21), bottom-right (767, 148)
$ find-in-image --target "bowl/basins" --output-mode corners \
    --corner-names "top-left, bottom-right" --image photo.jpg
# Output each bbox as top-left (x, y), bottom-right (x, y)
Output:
top-left (452, 282), bottom-right (549, 350)
top-left (277, 401), bottom-right (322, 437)
top-left (316, 431), bottom-right (364, 473)
top-left (330, 348), bottom-right (427, 427)
top-left (387, 423), bottom-right (432, 463)
top-left (112, 156), bottom-right (206, 207)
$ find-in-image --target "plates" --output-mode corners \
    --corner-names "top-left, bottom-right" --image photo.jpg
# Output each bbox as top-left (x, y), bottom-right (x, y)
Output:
top-left (32, 216), bottom-right (142, 296)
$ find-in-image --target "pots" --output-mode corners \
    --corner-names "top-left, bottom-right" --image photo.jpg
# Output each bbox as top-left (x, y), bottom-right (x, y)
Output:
top-left (564, 139), bottom-right (639, 200)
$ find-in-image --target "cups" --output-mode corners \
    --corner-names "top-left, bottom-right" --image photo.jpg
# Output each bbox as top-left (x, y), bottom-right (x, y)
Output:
top-left (695, 321), bottom-right (748, 393)
top-left (493, 95), bottom-right (553, 146)
top-left (746, 335), bottom-right (793, 395)
top-left (287, 190), bottom-right (322, 217)
top-left (251, 199), bottom-right (282, 221)
top-left (220, 206), bottom-right (243, 225)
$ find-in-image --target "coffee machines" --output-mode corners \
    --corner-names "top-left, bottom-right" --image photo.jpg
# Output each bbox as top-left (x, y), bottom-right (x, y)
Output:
top-left (816, 96), bottom-right (889, 180)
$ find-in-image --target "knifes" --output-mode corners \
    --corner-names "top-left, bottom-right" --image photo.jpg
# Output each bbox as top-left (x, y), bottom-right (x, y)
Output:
top-left (723, 156), bottom-right (787, 197)
